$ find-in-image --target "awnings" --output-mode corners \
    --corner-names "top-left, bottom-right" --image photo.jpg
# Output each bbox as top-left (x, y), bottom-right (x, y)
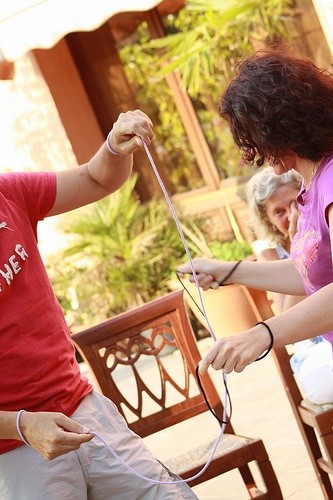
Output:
top-left (1, 0), bottom-right (175, 64)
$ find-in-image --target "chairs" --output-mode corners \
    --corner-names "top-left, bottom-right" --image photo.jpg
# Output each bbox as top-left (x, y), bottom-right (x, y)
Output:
top-left (70, 289), bottom-right (284, 500)
top-left (239, 259), bottom-right (333, 500)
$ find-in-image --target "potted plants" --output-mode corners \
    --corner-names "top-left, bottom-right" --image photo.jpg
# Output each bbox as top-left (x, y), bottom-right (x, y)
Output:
top-left (167, 242), bottom-right (254, 340)
top-left (104, 257), bottom-right (178, 355)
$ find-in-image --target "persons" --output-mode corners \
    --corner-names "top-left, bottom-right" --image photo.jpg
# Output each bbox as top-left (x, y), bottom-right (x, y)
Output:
top-left (176, 48), bottom-right (333, 377)
top-left (0, 109), bottom-right (200, 500)
top-left (243, 163), bottom-right (333, 406)
